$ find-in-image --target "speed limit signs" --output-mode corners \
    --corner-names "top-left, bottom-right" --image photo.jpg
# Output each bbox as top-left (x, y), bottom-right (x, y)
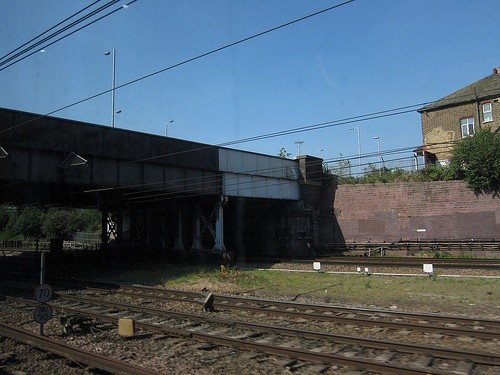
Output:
top-left (34, 283), bottom-right (53, 304)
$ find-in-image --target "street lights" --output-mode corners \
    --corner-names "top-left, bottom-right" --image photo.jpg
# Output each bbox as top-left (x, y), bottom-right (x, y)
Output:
top-left (372, 136), bottom-right (382, 176)
top-left (316, 149), bottom-right (324, 157)
top-left (165, 120), bottom-right (174, 136)
top-left (105, 48), bottom-right (115, 127)
top-left (350, 127), bottom-right (362, 177)
top-left (295, 141), bottom-right (304, 156)
